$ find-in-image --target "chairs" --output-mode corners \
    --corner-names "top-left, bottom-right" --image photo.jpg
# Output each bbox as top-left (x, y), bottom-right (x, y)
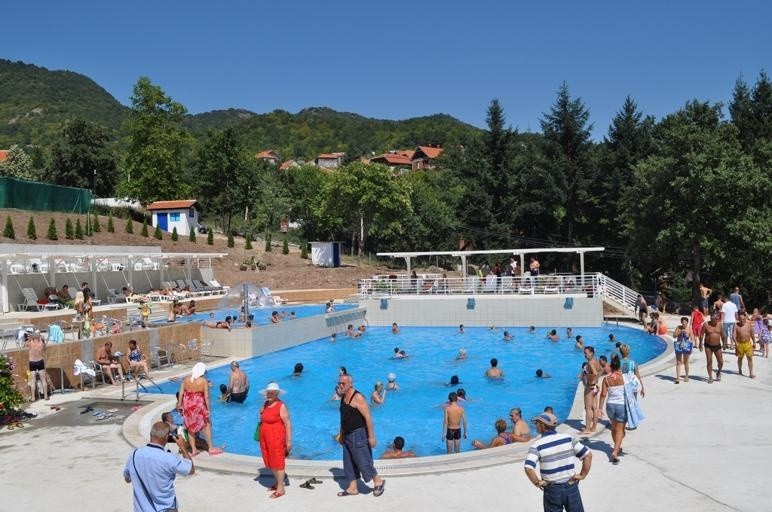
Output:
top-left (1, 315), bottom-right (214, 393)
top-left (17, 279), bottom-right (288, 311)
top-left (375, 274), bottom-right (577, 293)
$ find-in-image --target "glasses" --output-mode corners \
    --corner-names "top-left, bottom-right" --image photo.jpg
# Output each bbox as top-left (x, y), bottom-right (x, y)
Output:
top-left (338, 382), bottom-right (345, 385)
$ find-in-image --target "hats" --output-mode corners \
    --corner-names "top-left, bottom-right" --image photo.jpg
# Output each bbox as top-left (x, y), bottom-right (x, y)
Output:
top-left (387, 373), bottom-right (396, 381)
top-left (532, 413), bottom-right (558, 426)
top-left (259, 382), bottom-right (285, 396)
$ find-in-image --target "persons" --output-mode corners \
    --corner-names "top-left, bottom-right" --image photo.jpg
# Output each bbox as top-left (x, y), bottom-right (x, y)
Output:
top-left (357, 258), bottom-right (604, 298)
top-left (440, 324), bottom-right (646, 512)
top-left (25, 258), bottom-right (416, 511)
top-left (635, 281), bottom-right (772, 384)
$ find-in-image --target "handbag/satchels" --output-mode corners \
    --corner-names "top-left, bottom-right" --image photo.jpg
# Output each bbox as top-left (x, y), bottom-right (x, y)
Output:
top-left (674, 336), bottom-right (692, 354)
top-left (626, 360), bottom-right (642, 393)
top-left (253, 421), bottom-right (261, 441)
top-left (157, 508), bottom-right (178, 512)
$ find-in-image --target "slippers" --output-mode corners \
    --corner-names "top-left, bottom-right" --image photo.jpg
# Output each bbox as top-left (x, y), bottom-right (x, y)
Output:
top-left (617, 448), bottom-right (624, 456)
top-left (708, 378), bottom-right (712, 383)
top-left (299, 481), bottom-right (315, 489)
top-left (271, 491), bottom-right (285, 498)
top-left (373, 480), bottom-right (385, 497)
top-left (269, 484), bottom-right (284, 491)
top-left (190, 449), bottom-right (200, 457)
top-left (716, 371), bottom-right (721, 382)
top-left (337, 490), bottom-right (359, 496)
top-left (613, 459), bottom-right (620, 465)
top-left (309, 478), bottom-right (323, 484)
top-left (208, 448), bottom-right (224, 454)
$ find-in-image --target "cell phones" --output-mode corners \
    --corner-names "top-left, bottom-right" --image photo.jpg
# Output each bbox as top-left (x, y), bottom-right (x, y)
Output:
top-left (167, 435), bottom-right (178, 442)
